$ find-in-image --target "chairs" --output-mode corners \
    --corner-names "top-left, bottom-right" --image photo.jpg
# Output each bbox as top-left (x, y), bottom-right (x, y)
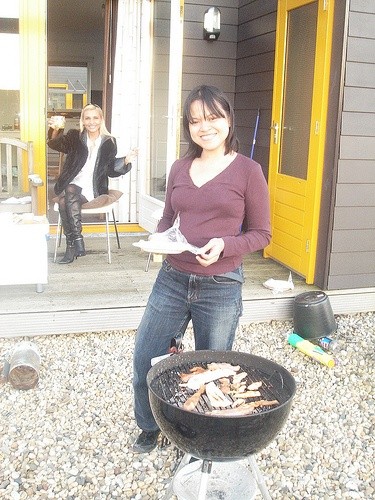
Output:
top-left (0, 138), bottom-right (44, 215)
top-left (47, 146), bottom-right (64, 182)
top-left (52, 188), bottom-right (121, 264)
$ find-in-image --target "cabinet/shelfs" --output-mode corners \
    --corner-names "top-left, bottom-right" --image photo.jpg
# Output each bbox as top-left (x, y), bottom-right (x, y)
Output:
top-left (0, 127), bottom-right (80, 167)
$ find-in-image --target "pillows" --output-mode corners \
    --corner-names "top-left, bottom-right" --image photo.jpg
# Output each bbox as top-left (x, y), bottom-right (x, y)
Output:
top-left (51, 190), bottom-right (124, 209)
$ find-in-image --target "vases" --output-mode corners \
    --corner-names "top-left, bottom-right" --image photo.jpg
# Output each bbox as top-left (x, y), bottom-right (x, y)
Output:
top-left (293, 291), bottom-right (339, 340)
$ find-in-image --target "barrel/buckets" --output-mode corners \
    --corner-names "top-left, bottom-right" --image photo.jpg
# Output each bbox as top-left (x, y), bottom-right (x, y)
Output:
top-left (9, 340), bottom-right (43, 389)
top-left (294, 290), bottom-right (337, 341)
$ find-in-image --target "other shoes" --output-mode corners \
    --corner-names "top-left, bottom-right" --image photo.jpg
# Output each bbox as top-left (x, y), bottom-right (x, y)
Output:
top-left (134, 432), bottom-right (160, 453)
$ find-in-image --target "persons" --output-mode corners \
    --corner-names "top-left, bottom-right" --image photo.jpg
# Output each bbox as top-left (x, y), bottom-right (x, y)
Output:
top-left (48, 105), bottom-right (140, 266)
top-left (133, 85), bottom-right (272, 456)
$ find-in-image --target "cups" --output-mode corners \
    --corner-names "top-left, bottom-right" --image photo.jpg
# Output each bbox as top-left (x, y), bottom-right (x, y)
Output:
top-left (52, 116), bottom-right (65, 127)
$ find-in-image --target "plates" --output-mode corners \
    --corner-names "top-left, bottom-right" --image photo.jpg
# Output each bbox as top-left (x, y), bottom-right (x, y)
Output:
top-left (133, 240), bottom-right (191, 254)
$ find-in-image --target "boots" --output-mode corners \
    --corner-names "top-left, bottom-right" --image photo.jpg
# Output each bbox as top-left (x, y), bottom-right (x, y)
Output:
top-left (58, 206), bottom-right (78, 264)
top-left (64, 192), bottom-right (85, 257)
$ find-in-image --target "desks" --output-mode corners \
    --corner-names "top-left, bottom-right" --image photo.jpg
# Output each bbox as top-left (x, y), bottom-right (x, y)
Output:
top-left (0, 229), bottom-right (49, 293)
